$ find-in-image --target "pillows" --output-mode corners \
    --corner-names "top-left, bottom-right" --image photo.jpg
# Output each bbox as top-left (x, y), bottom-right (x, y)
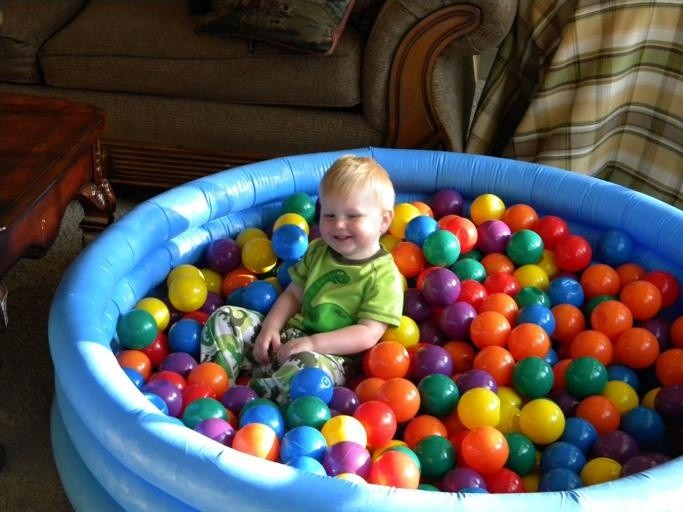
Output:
top-left (186, 1), bottom-right (356, 58)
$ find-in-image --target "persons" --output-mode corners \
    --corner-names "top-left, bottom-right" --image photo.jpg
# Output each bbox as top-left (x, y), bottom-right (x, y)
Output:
top-left (199, 154), bottom-right (405, 413)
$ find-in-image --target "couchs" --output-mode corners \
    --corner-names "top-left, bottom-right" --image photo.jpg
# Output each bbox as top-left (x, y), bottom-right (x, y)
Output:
top-left (0, 0), bottom-right (524, 213)
